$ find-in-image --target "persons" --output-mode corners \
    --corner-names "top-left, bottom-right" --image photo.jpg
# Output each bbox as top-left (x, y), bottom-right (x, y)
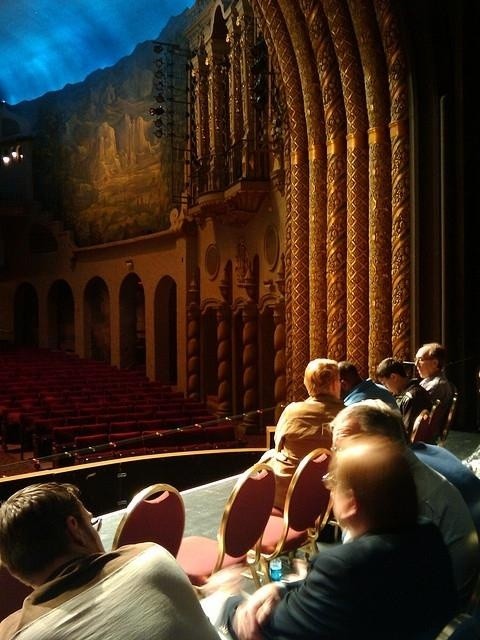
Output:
top-left (332, 397), bottom-right (480, 613)
top-left (213, 432), bottom-right (461, 640)
top-left (0, 482), bottom-right (222, 639)
top-left (413, 342), bottom-right (453, 422)
top-left (255, 358), bottom-right (344, 511)
top-left (333, 361), bottom-right (399, 415)
top-left (374, 355), bottom-right (432, 437)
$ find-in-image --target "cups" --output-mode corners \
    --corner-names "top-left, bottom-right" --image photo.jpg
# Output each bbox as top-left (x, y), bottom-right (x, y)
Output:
top-left (268, 559), bottom-right (282, 581)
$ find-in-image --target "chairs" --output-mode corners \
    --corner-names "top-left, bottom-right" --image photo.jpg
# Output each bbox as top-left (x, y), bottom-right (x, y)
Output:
top-left (110, 449), bottom-right (336, 588)
top-left (411, 382), bottom-right (458, 446)
top-left (0, 392), bottom-right (246, 463)
